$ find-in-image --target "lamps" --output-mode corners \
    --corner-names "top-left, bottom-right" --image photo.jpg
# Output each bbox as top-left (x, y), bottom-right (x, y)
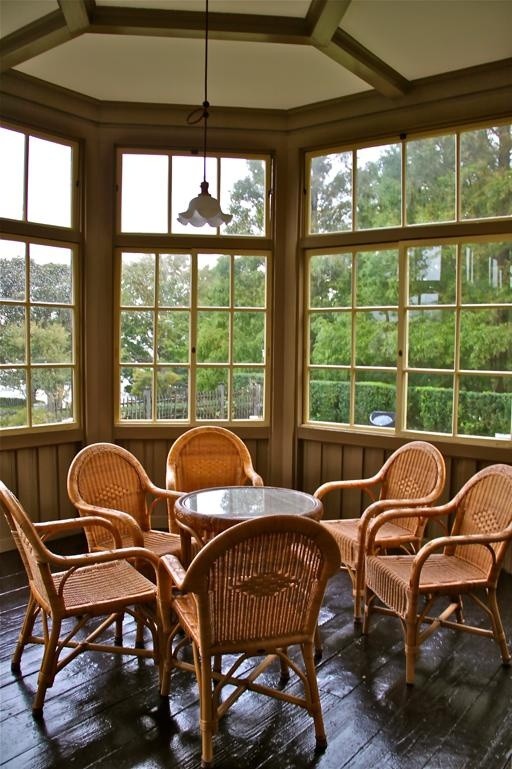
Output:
top-left (177, 0), bottom-right (233, 227)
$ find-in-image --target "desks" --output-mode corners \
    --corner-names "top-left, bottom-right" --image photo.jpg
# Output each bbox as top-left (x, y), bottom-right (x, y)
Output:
top-left (173, 486), bottom-right (324, 659)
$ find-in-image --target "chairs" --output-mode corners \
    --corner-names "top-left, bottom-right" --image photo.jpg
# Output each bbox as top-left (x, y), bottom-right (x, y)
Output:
top-left (66, 441), bottom-right (204, 646)
top-left (312, 441), bottom-right (446, 624)
top-left (0, 481), bottom-right (170, 714)
top-left (158, 511), bottom-right (342, 769)
top-left (166, 426), bottom-right (264, 536)
top-left (351, 464), bottom-right (512, 684)
